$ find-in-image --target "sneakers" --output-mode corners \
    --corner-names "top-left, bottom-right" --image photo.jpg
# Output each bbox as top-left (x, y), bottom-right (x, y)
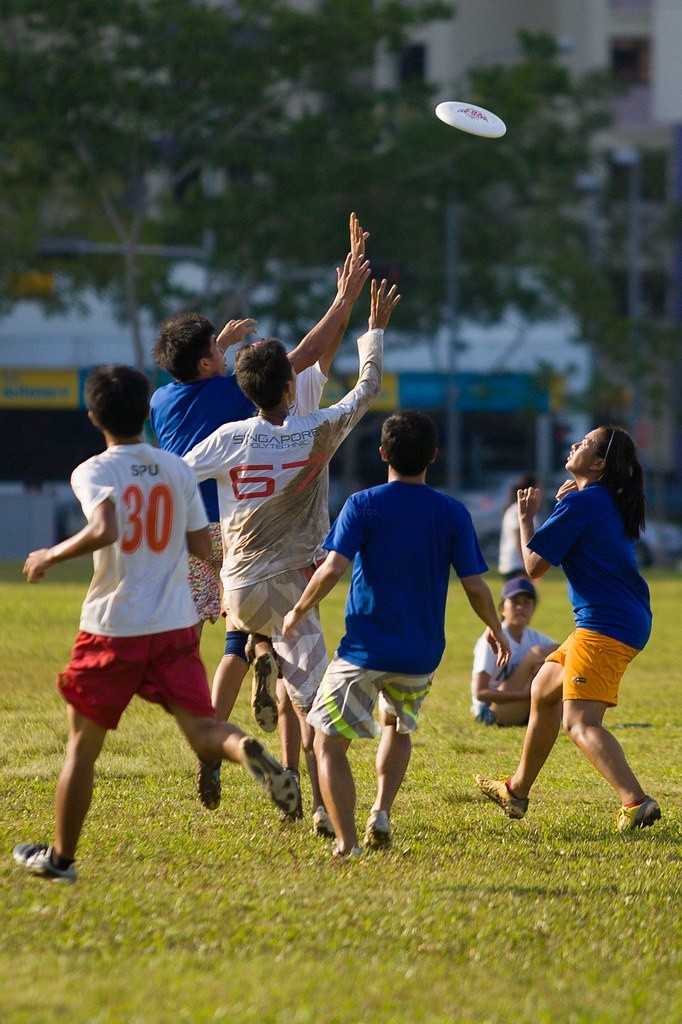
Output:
top-left (614, 794), bottom-right (661, 832)
top-left (12, 844), bottom-right (76, 883)
top-left (476, 773), bottom-right (529, 820)
top-left (239, 736), bottom-right (298, 814)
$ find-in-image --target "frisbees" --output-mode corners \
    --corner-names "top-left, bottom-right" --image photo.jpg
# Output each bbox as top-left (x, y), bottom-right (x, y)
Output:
top-left (434, 100), bottom-right (507, 139)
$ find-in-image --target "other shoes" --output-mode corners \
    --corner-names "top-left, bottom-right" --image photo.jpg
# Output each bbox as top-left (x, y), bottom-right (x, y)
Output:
top-left (251, 651), bottom-right (280, 733)
top-left (197, 758), bottom-right (221, 809)
top-left (276, 790), bottom-right (304, 823)
top-left (363, 810), bottom-right (391, 849)
top-left (322, 844), bottom-right (361, 859)
top-left (312, 805), bottom-right (336, 839)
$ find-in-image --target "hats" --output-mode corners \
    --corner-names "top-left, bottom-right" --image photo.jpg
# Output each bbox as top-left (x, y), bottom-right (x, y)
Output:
top-left (503, 578), bottom-right (537, 600)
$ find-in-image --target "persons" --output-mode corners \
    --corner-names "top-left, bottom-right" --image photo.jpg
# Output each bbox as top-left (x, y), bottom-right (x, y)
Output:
top-left (283, 410), bottom-right (511, 858)
top-left (477, 426), bottom-right (661, 832)
top-left (147, 213), bottom-right (402, 838)
top-left (11, 364), bottom-right (301, 884)
top-left (498, 475), bottom-right (543, 582)
top-left (471, 578), bottom-right (561, 727)
